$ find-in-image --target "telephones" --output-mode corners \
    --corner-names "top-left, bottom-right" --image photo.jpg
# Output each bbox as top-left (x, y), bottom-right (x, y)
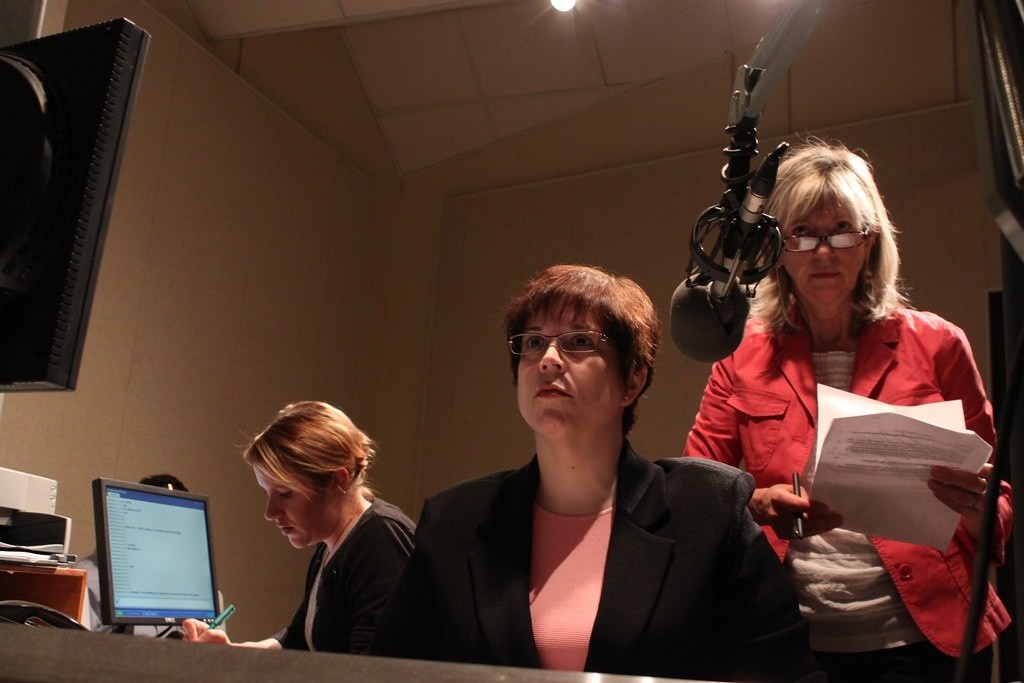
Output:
top-left (0, 600), bottom-right (92, 632)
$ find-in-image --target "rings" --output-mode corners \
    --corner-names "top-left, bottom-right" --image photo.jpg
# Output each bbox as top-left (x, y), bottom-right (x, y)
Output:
top-left (978, 478), bottom-right (988, 496)
top-left (970, 494), bottom-right (978, 509)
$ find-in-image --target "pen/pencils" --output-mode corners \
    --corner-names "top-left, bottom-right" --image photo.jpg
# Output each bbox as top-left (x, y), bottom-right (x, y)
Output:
top-left (790, 471), bottom-right (805, 539)
top-left (208, 604), bottom-right (235, 630)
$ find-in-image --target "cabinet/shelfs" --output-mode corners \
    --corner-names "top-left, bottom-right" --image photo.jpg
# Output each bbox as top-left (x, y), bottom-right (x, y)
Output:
top-left (0, 566), bottom-right (88, 624)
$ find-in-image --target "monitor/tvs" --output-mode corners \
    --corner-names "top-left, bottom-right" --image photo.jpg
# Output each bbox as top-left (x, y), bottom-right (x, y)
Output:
top-left (93, 476), bottom-right (222, 635)
top-left (0, 17), bottom-right (152, 392)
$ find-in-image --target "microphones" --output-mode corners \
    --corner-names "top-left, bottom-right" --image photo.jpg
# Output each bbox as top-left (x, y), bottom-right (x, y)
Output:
top-left (666, 142), bottom-right (790, 364)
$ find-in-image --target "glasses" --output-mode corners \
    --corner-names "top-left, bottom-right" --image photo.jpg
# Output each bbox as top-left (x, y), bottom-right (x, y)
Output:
top-left (781, 224), bottom-right (870, 252)
top-left (506, 330), bottom-right (617, 355)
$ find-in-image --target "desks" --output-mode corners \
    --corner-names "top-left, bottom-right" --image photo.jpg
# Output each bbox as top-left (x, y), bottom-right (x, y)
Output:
top-left (0, 620), bottom-right (682, 683)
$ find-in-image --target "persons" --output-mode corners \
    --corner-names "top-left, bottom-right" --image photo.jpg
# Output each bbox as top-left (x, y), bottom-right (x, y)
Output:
top-left (182, 401), bottom-right (416, 655)
top-left (375, 264), bottom-right (805, 683)
top-left (76, 474), bottom-right (188, 637)
top-left (683, 148), bottom-right (1012, 683)
